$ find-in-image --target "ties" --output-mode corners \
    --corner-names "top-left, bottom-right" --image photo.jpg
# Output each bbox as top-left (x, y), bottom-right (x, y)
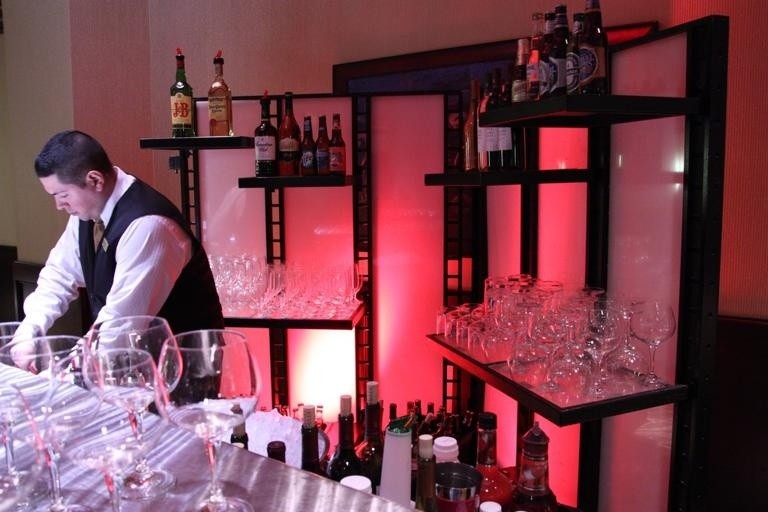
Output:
top-left (93, 217), bottom-right (104, 255)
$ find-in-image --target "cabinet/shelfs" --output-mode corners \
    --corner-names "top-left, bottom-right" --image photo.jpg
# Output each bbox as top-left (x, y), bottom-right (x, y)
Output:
top-left (137, 91), bottom-right (373, 427)
top-left (426, 13), bottom-right (730, 512)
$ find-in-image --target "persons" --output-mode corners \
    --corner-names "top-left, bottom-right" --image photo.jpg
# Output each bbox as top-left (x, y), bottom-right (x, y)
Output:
top-left (9, 129), bottom-right (224, 417)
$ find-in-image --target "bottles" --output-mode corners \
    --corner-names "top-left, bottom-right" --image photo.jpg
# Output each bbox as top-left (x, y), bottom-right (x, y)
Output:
top-left (254, 97), bottom-right (278, 177)
top-left (170, 54), bottom-right (195, 138)
top-left (315, 115), bottom-right (330, 176)
top-left (328, 112), bottom-right (346, 177)
top-left (208, 57), bottom-right (233, 135)
top-left (230, 381), bottom-right (384, 495)
top-left (277, 91), bottom-right (301, 176)
top-left (299, 115), bottom-right (316, 177)
top-left (462, 66), bottom-right (523, 171)
top-left (512, 0), bottom-right (612, 101)
top-left (380, 400), bottom-right (557, 511)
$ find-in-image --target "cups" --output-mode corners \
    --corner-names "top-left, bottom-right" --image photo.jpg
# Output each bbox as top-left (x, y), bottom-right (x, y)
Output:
top-left (435, 274), bottom-right (532, 359)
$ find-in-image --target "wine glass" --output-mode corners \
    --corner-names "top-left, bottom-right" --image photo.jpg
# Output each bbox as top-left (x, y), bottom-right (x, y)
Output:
top-left (493, 281), bottom-right (674, 407)
top-left (207, 249), bottom-right (363, 323)
top-left (1, 316), bottom-right (184, 510)
top-left (159, 330), bottom-right (258, 511)
